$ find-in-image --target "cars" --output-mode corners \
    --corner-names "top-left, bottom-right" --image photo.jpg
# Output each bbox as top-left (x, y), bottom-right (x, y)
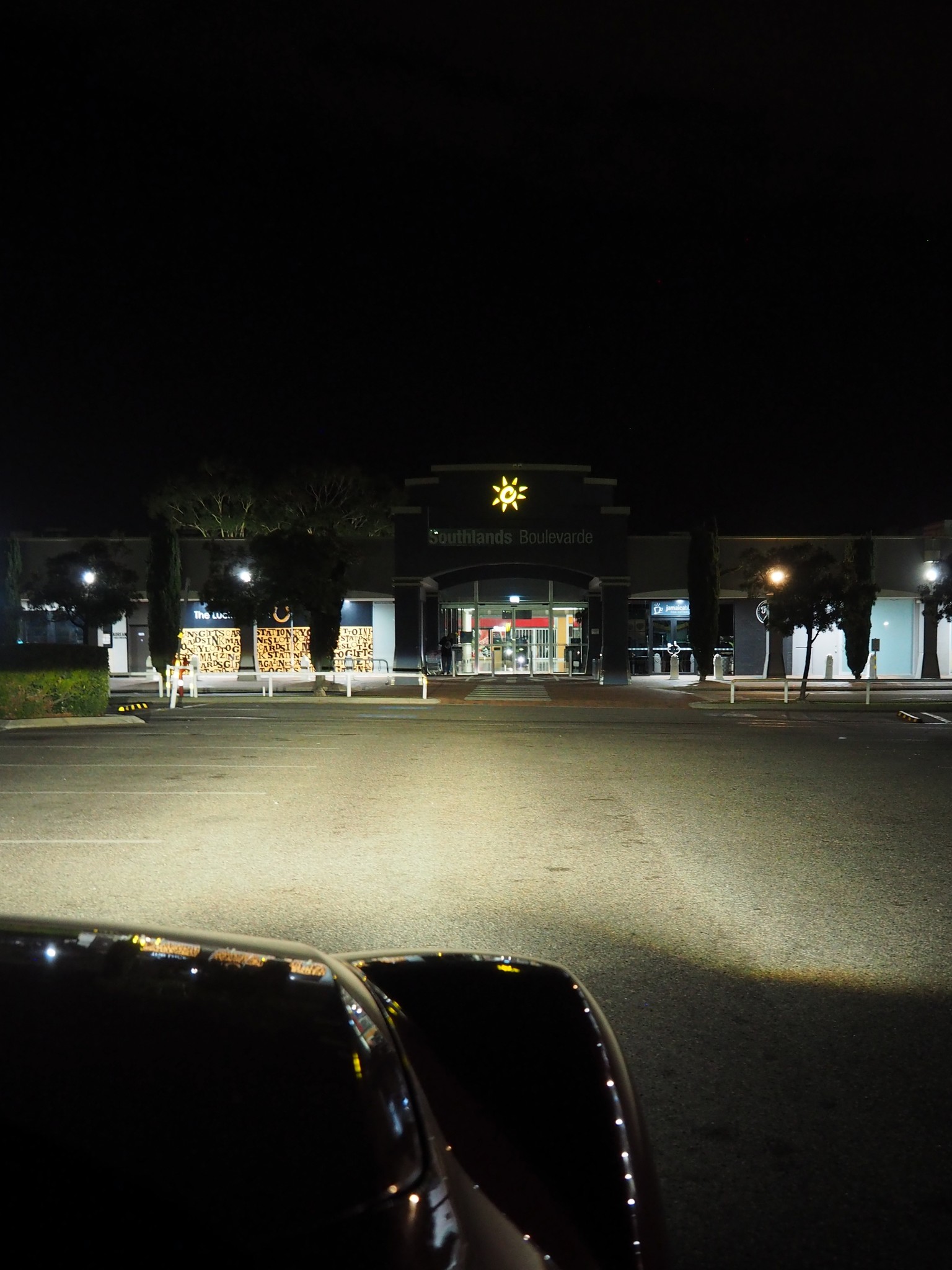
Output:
top-left (0, 915), bottom-right (660, 1269)
